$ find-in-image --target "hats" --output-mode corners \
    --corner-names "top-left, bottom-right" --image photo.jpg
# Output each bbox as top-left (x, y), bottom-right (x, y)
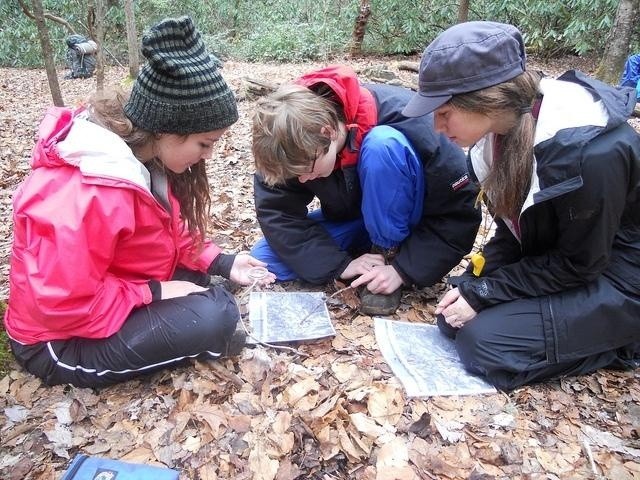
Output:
top-left (402, 21), bottom-right (525, 117)
top-left (124, 16), bottom-right (239, 133)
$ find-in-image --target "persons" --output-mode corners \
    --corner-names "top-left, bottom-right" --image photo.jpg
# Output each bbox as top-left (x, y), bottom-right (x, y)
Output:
top-left (2, 13), bottom-right (277, 387)
top-left (619, 52), bottom-right (640, 88)
top-left (402, 21), bottom-right (640, 391)
top-left (250, 65), bottom-right (482, 316)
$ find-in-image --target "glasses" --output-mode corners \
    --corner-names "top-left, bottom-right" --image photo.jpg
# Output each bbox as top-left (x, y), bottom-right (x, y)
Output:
top-left (291, 145), bottom-right (318, 175)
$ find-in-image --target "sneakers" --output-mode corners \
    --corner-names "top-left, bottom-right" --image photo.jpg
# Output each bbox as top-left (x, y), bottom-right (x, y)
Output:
top-left (360, 248), bottom-right (401, 315)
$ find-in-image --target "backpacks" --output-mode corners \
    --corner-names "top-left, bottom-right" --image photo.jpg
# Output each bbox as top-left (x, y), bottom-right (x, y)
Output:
top-left (66, 35), bottom-right (95, 77)
